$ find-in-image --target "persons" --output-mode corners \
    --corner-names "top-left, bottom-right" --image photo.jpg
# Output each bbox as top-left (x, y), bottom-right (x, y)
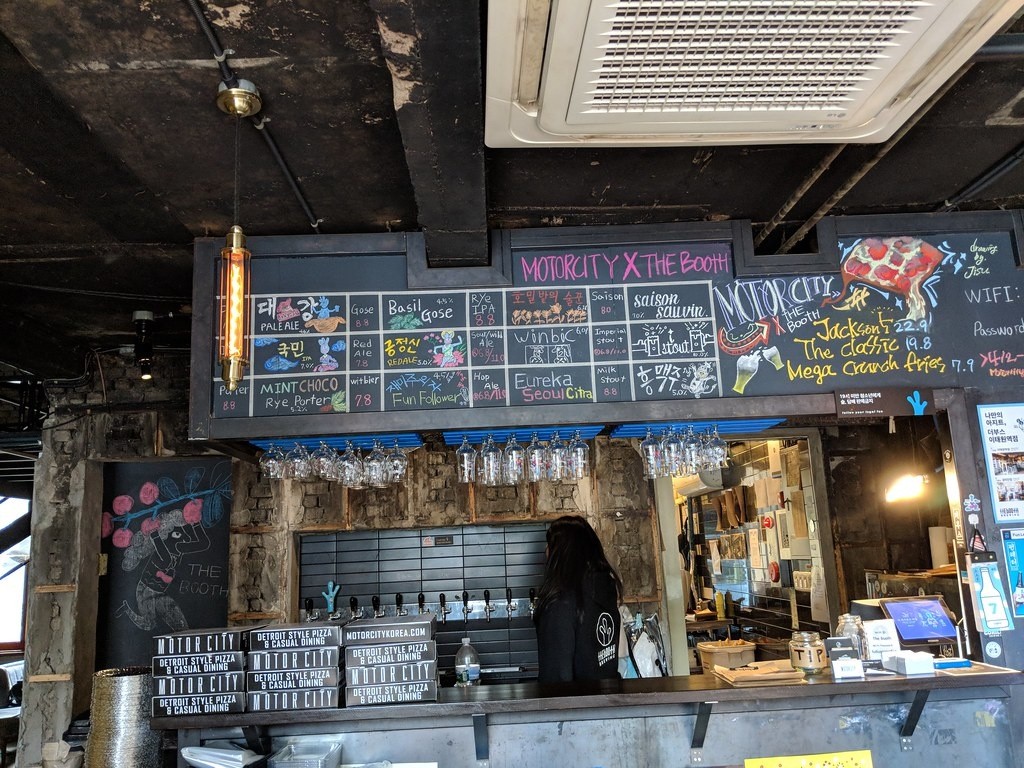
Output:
top-left (535, 516), bottom-right (623, 684)
top-left (678, 517), bottom-right (717, 614)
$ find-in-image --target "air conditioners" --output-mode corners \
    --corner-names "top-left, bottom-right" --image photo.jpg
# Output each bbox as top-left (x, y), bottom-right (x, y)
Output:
top-left (678, 468), bottom-right (723, 498)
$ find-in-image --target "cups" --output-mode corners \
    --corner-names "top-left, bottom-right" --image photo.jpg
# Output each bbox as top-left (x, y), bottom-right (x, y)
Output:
top-left (256, 425), bottom-right (729, 486)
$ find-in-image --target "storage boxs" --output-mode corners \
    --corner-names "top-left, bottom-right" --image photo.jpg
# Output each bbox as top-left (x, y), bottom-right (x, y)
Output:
top-left (151, 614), bottom-right (439, 718)
top-left (696, 639), bottom-right (756, 676)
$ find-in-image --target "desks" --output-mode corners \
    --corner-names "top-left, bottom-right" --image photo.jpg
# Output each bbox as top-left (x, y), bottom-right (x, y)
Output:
top-left (684, 618), bottom-right (735, 642)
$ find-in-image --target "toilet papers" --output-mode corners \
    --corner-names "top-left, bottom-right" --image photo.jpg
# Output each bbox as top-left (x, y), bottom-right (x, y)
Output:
top-left (928, 527), bottom-right (953, 569)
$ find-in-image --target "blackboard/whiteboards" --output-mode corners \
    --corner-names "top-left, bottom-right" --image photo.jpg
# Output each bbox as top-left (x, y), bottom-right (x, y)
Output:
top-left (189, 211), bottom-right (1024, 438)
top-left (92, 455), bottom-right (232, 673)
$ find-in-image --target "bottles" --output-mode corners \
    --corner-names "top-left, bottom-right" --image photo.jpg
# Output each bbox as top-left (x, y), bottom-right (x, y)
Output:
top-left (1015, 571), bottom-right (1024, 604)
top-left (455, 636), bottom-right (482, 687)
top-left (788, 631), bottom-right (830, 676)
top-left (836, 614), bottom-right (868, 663)
top-left (715, 589), bottom-right (725, 622)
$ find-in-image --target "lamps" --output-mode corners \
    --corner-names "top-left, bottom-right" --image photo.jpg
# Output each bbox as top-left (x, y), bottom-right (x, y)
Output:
top-left (217, 77), bottom-right (263, 396)
